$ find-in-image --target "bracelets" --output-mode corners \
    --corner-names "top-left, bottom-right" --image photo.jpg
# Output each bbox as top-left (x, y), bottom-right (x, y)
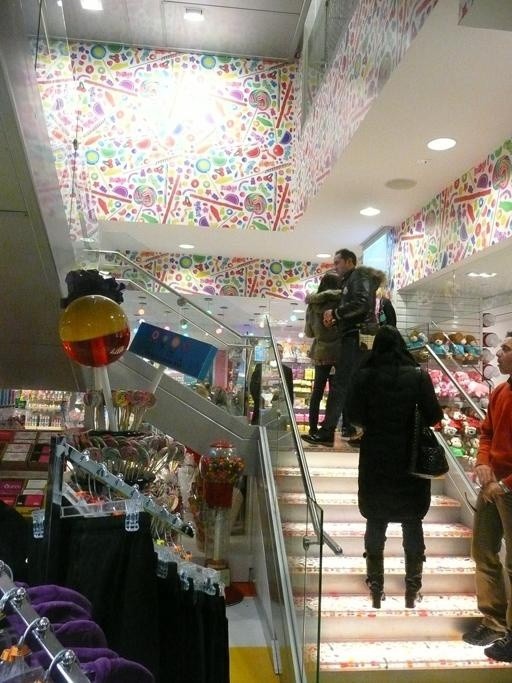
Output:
top-left (498, 480), bottom-right (510, 494)
top-left (332, 307), bottom-right (342, 321)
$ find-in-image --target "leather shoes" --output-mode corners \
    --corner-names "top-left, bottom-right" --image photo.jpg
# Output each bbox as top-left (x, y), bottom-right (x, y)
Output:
top-left (341, 427), bottom-right (364, 448)
top-left (300, 428), bottom-right (335, 447)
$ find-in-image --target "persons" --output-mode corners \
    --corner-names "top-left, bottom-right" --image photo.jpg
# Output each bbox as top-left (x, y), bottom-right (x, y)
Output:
top-left (462, 329), bottom-right (512, 663)
top-left (250, 343), bottom-right (294, 425)
top-left (297, 271), bottom-right (347, 435)
top-left (375, 287), bottom-right (397, 328)
top-left (341, 324), bottom-right (447, 609)
top-left (301, 247), bottom-right (388, 448)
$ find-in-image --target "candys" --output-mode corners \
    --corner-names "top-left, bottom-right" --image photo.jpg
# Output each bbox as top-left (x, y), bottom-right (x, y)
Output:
top-left (201, 455), bottom-right (245, 484)
top-left (65, 269), bottom-right (126, 430)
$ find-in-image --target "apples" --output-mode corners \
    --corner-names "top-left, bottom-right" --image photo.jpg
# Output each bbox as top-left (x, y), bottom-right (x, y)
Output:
top-left (58, 294), bottom-right (140, 432)
top-left (67, 388), bottom-right (186, 481)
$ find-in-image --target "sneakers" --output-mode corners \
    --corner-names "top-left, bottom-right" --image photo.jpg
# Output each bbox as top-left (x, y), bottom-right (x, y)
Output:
top-left (462, 622), bottom-right (512, 663)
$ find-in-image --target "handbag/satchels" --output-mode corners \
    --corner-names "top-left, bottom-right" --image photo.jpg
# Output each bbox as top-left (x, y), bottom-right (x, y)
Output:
top-left (358, 326), bottom-right (376, 351)
top-left (407, 425), bottom-right (450, 480)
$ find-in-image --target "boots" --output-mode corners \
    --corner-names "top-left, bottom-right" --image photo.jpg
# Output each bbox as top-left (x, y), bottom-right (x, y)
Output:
top-left (363, 552), bottom-right (385, 609)
top-left (404, 554), bottom-right (426, 608)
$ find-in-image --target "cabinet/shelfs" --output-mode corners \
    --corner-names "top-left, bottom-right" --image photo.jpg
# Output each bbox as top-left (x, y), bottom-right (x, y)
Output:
top-left (0, 429), bottom-right (67, 520)
top-left (282, 341), bottom-right (330, 424)
